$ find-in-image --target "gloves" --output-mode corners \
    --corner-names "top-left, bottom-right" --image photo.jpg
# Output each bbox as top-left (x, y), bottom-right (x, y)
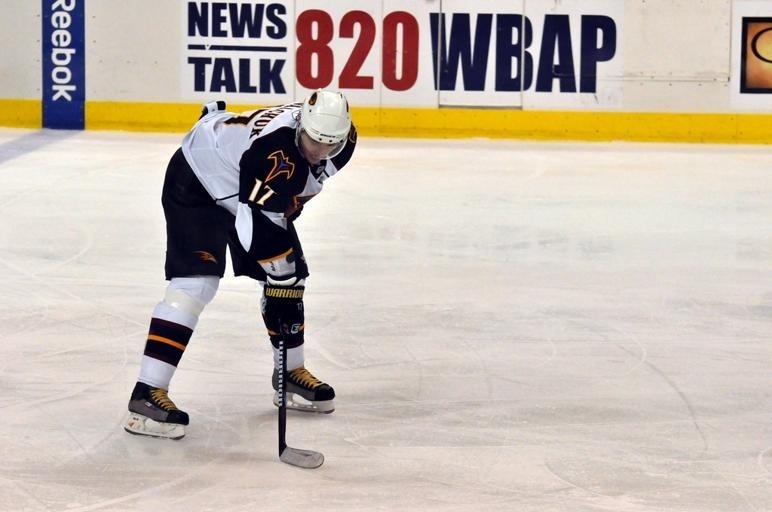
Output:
top-left (259, 280), bottom-right (306, 340)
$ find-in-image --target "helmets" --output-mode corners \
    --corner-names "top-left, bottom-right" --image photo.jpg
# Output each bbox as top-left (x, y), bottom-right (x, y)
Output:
top-left (293, 87), bottom-right (353, 162)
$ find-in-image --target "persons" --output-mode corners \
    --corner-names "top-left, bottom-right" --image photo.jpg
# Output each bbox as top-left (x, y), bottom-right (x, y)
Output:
top-left (127, 89), bottom-right (357, 424)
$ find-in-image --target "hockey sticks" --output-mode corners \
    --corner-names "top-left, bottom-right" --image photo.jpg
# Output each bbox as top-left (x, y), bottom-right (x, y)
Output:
top-left (278, 331), bottom-right (324, 468)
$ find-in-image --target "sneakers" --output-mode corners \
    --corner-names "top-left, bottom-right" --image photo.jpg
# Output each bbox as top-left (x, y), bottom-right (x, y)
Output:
top-left (127, 381), bottom-right (190, 425)
top-left (271, 366), bottom-right (335, 403)
